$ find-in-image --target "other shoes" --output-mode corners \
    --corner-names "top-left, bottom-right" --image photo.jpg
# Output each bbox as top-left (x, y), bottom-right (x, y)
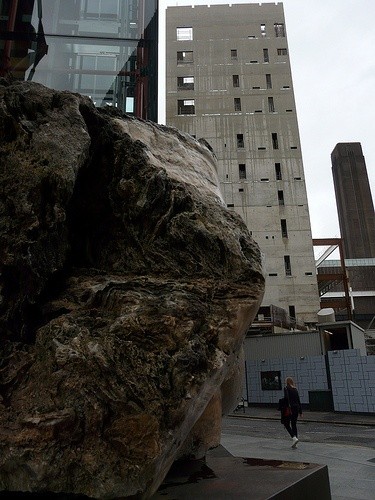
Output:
top-left (291, 437), bottom-right (299, 449)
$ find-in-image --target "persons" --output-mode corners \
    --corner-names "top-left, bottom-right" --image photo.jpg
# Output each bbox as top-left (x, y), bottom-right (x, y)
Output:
top-left (279, 378), bottom-right (302, 448)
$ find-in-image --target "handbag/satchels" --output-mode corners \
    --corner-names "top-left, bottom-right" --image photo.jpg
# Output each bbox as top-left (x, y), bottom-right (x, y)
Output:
top-left (284, 402), bottom-right (292, 416)
